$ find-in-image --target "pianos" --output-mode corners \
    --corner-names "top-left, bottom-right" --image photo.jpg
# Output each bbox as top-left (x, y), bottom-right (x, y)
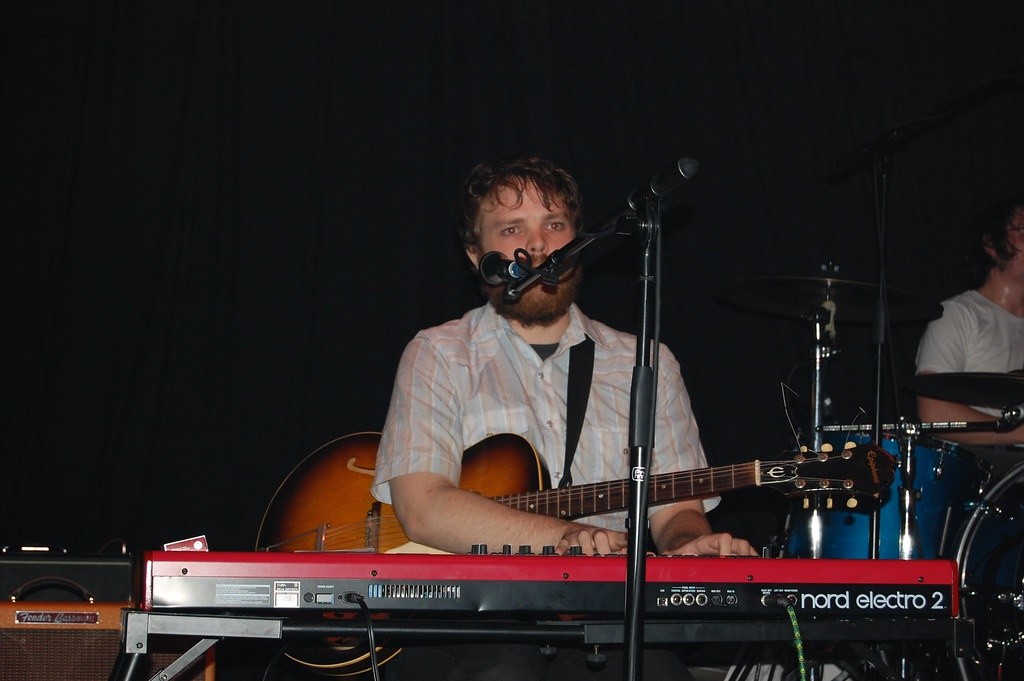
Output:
top-left (126, 543), bottom-right (963, 624)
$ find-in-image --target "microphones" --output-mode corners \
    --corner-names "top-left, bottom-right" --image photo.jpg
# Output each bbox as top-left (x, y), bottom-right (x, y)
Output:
top-left (480, 250), bottom-right (526, 288)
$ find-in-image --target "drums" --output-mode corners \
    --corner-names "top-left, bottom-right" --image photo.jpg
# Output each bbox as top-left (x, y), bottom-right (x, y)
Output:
top-left (783, 430), bottom-right (994, 564)
top-left (942, 462), bottom-right (1024, 681)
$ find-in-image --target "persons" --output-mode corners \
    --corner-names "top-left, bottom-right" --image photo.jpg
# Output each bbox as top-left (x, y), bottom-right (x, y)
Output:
top-left (915, 193), bottom-right (1024, 446)
top-left (370, 152), bottom-right (764, 681)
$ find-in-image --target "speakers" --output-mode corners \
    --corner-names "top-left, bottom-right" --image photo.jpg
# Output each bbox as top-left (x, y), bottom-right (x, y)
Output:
top-left (0, 601), bottom-right (216, 681)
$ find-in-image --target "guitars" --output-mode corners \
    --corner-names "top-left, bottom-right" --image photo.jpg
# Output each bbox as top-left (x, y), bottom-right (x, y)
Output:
top-left (256, 430), bottom-right (894, 678)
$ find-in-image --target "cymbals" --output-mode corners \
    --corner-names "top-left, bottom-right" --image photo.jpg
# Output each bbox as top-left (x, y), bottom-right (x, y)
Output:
top-left (713, 274), bottom-right (944, 326)
